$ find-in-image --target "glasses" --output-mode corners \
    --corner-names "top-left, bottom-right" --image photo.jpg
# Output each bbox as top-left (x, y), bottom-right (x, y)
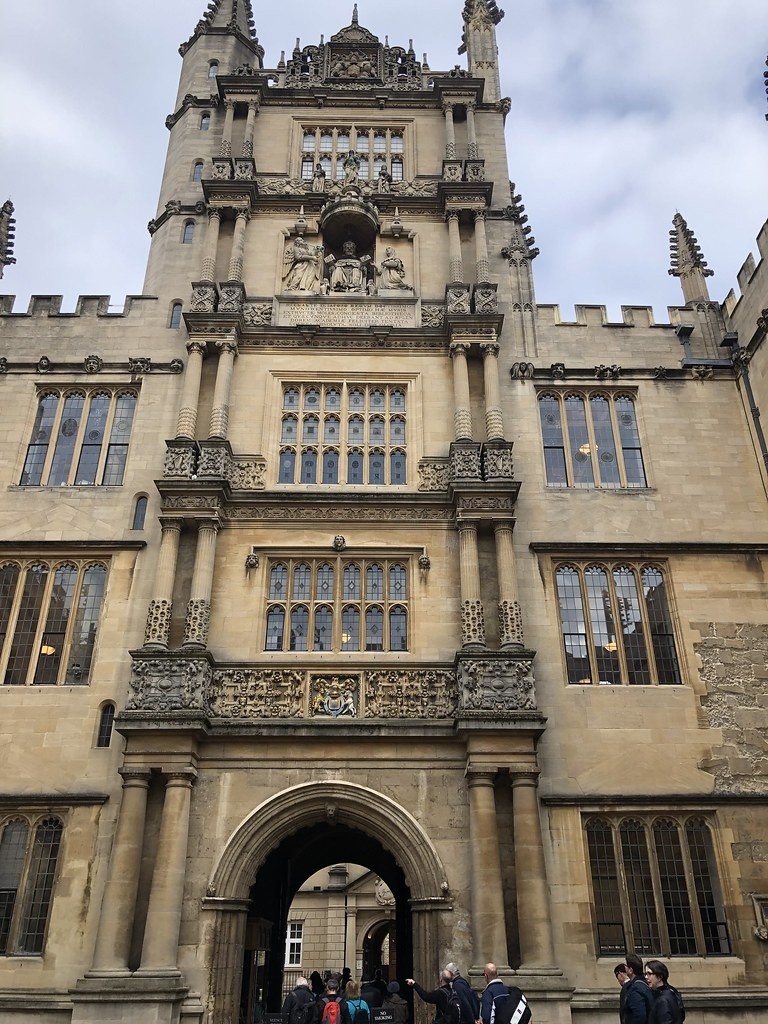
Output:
top-left (616, 973), bottom-right (620, 979)
top-left (623, 963), bottom-right (627, 968)
top-left (644, 972), bottom-right (654, 976)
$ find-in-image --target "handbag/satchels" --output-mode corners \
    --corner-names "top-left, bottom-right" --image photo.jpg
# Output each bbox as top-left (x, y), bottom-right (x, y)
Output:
top-left (494, 987), bottom-right (532, 1024)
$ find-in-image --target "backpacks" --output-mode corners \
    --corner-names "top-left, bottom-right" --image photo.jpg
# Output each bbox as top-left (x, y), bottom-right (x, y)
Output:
top-left (322, 997), bottom-right (343, 1024)
top-left (438, 988), bottom-right (464, 1024)
top-left (291, 992), bottom-right (316, 1024)
top-left (348, 999), bottom-right (369, 1024)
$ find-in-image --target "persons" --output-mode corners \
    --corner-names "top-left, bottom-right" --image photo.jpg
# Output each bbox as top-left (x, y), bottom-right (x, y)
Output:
top-left (312, 163), bottom-right (326, 191)
top-left (378, 164), bottom-right (393, 193)
top-left (331, 241), bottom-right (368, 293)
top-left (245, 553), bottom-right (259, 568)
top-left (480, 963), bottom-right (511, 1024)
top-left (342, 149), bottom-right (360, 186)
top-left (331, 54), bottom-right (377, 77)
top-left (370, 246), bottom-right (412, 290)
top-left (281, 967), bottom-right (409, 1024)
top-left (282, 237), bottom-right (324, 291)
top-left (404, 962), bottom-right (483, 1024)
top-left (332, 535), bottom-right (346, 550)
top-left (614, 954), bottom-right (685, 1024)
top-left (417, 555), bottom-right (430, 569)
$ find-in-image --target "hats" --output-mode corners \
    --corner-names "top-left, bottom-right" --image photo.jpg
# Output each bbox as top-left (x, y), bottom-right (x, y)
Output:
top-left (387, 981), bottom-right (400, 993)
top-left (295, 977), bottom-right (308, 986)
